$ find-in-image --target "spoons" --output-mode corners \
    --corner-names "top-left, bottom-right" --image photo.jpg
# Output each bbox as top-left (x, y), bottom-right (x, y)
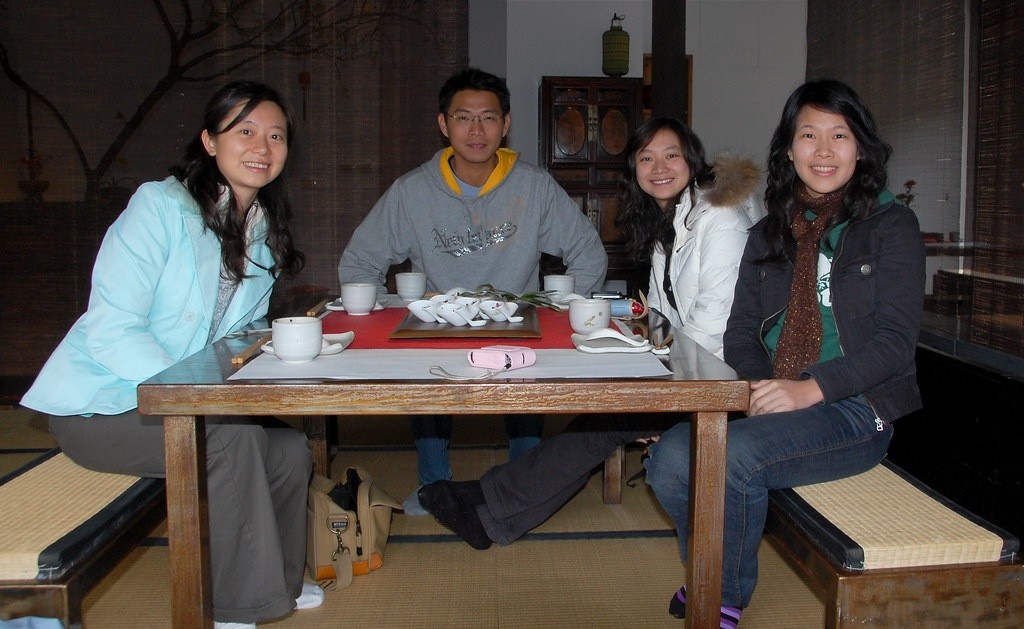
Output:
top-left (586, 328), bottom-right (649, 347)
top-left (561, 293), bottom-right (585, 302)
top-left (453, 309), bottom-right (487, 326)
top-left (495, 303), bottom-right (524, 323)
top-left (423, 308), bottom-right (448, 323)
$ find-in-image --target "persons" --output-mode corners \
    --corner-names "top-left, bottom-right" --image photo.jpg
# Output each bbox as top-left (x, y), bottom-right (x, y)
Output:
top-left (18, 83), bottom-right (325, 629)
top-left (648, 80), bottom-right (930, 629)
top-left (416, 118), bottom-right (767, 550)
top-left (338, 70), bottom-right (608, 518)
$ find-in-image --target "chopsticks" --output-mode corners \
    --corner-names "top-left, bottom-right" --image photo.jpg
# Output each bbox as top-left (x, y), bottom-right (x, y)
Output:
top-left (231, 335), bottom-right (270, 364)
top-left (307, 297), bottom-right (329, 316)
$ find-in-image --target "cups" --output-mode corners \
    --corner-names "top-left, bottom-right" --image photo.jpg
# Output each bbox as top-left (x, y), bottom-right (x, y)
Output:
top-left (569, 299), bottom-right (610, 335)
top-left (949, 232), bottom-right (960, 242)
top-left (544, 275), bottom-right (575, 304)
top-left (272, 317), bottom-right (322, 363)
top-left (341, 283), bottom-right (377, 316)
top-left (396, 273), bottom-right (427, 302)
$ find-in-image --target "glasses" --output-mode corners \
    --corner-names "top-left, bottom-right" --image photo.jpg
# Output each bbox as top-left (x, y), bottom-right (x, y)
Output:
top-left (446, 112), bottom-right (503, 126)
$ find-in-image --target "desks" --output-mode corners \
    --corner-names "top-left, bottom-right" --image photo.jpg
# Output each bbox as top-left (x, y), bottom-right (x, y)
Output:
top-left (137, 295), bottom-right (749, 629)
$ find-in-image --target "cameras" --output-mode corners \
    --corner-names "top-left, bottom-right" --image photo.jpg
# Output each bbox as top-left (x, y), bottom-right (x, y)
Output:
top-left (468, 347), bottom-right (536, 370)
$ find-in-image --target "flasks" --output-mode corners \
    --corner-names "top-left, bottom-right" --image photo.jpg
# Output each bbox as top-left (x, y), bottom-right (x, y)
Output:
top-left (610, 290), bottom-right (649, 320)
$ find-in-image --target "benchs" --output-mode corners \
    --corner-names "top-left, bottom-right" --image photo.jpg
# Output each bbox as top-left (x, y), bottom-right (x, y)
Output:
top-left (0, 447), bottom-right (168, 629)
top-left (767, 459), bottom-right (1019, 629)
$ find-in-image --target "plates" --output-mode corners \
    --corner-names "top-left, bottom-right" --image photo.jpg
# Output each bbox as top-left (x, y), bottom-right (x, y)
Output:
top-left (571, 333), bottom-right (652, 354)
top-left (261, 331), bottom-right (355, 355)
top-left (325, 299), bottom-right (391, 311)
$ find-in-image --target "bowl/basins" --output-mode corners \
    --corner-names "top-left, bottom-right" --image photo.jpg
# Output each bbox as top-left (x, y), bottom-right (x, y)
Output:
top-left (437, 301), bottom-right (479, 326)
top-left (479, 301), bottom-right (518, 322)
top-left (406, 300), bottom-right (439, 323)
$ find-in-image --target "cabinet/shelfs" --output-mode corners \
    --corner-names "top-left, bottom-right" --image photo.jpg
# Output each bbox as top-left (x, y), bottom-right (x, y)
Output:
top-left (537, 75), bottom-right (644, 292)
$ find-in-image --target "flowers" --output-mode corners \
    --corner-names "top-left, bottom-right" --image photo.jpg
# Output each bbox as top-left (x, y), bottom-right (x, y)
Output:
top-left (896, 179), bottom-right (917, 209)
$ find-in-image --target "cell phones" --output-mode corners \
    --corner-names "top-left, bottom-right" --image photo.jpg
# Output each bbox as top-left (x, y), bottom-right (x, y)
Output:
top-left (592, 290), bottom-right (622, 299)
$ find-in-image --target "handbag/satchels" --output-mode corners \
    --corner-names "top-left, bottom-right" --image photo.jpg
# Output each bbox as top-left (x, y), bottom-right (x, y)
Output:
top-left (308, 464), bottom-right (404, 582)
